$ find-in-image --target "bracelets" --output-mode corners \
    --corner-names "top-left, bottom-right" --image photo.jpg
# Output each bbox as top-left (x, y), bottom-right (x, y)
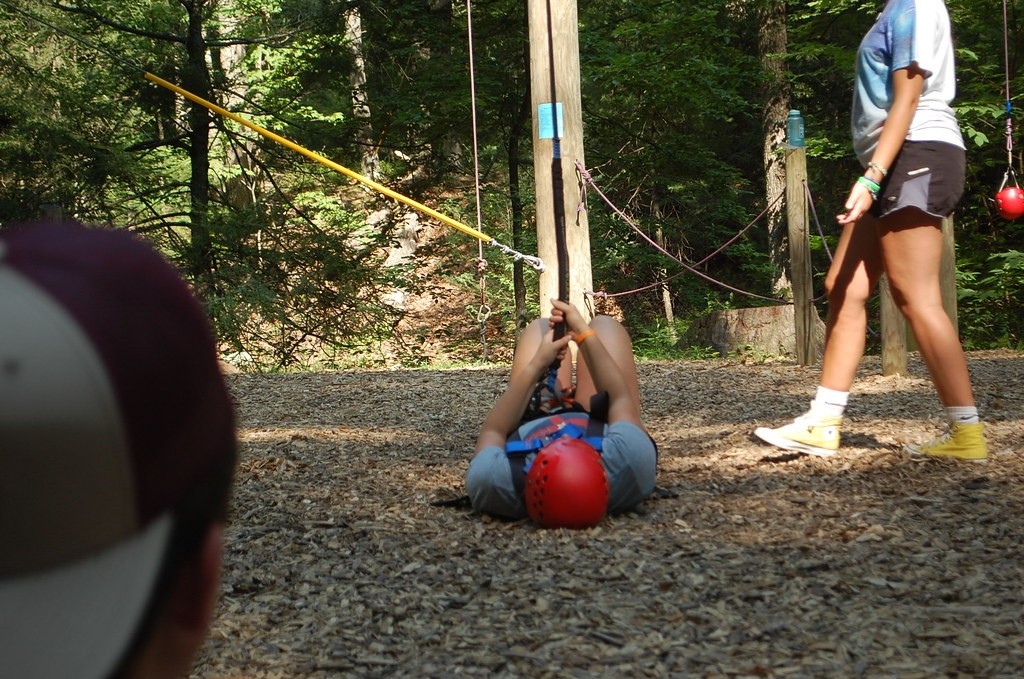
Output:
top-left (855, 161), bottom-right (887, 200)
top-left (572, 330), bottom-right (597, 345)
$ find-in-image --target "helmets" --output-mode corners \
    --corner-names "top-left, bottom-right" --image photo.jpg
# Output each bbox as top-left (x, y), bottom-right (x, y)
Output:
top-left (994, 187), bottom-right (1024, 220)
top-left (525, 438), bottom-right (609, 530)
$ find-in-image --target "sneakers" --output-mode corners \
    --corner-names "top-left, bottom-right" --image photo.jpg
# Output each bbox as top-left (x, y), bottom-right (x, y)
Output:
top-left (754, 418), bottom-right (840, 456)
top-left (903, 422), bottom-right (987, 462)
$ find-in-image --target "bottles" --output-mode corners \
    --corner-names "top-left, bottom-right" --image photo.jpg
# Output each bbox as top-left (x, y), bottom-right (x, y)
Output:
top-left (786, 110), bottom-right (804, 146)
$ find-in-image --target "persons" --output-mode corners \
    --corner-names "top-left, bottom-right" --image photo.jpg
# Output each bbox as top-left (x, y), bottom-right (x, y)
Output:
top-left (0, 220), bottom-right (243, 679)
top-left (461, 298), bottom-right (660, 531)
top-left (753, 0), bottom-right (988, 464)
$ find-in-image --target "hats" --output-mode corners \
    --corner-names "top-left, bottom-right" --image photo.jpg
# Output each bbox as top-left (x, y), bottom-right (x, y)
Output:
top-left (0, 223), bottom-right (217, 679)
